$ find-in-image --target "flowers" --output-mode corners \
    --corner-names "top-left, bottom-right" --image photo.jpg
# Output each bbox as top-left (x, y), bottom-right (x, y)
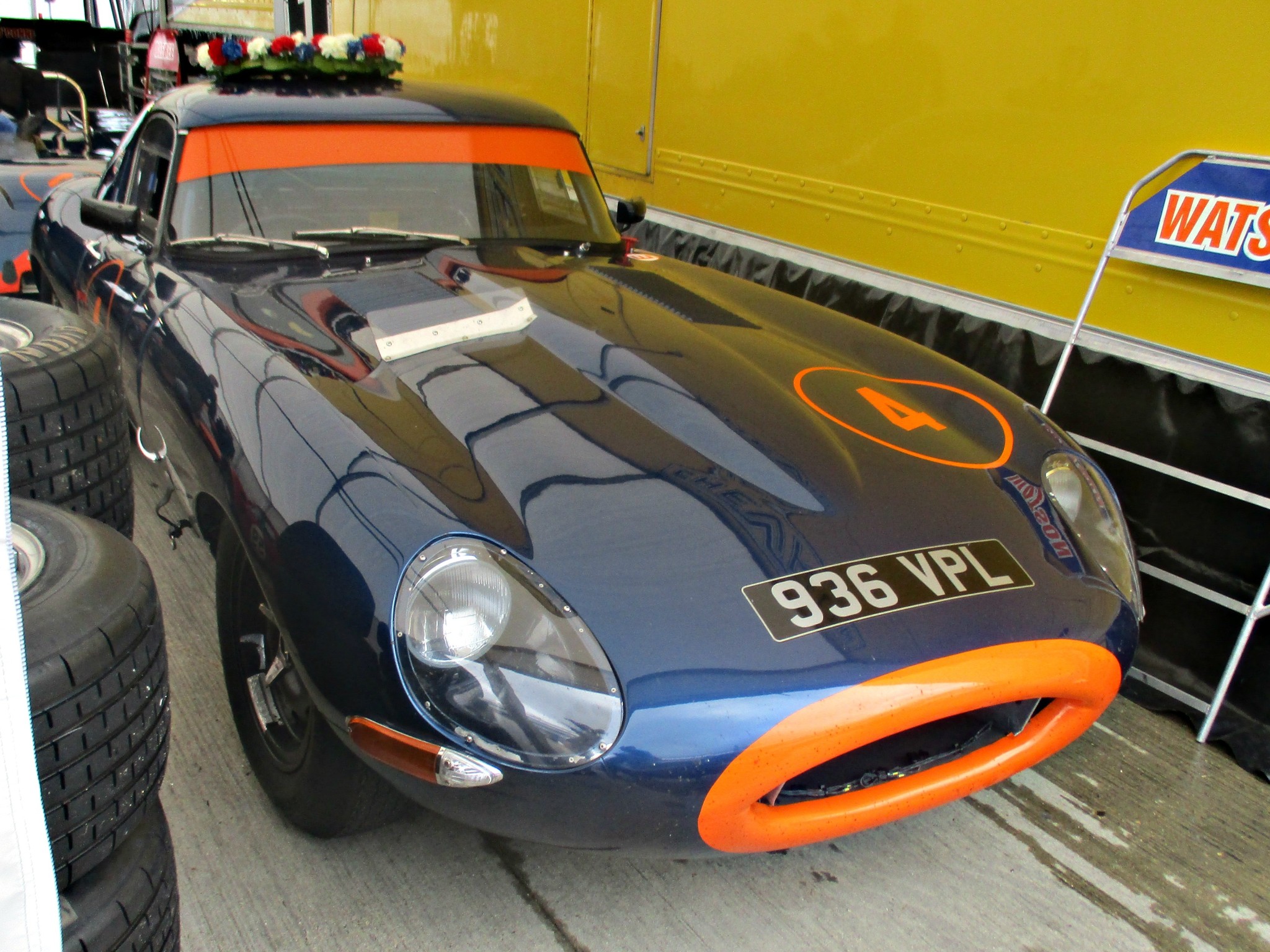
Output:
top-left (195, 30), bottom-right (409, 84)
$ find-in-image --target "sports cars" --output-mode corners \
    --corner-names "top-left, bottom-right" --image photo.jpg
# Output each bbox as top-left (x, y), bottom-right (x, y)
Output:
top-left (31, 72), bottom-right (1147, 852)
